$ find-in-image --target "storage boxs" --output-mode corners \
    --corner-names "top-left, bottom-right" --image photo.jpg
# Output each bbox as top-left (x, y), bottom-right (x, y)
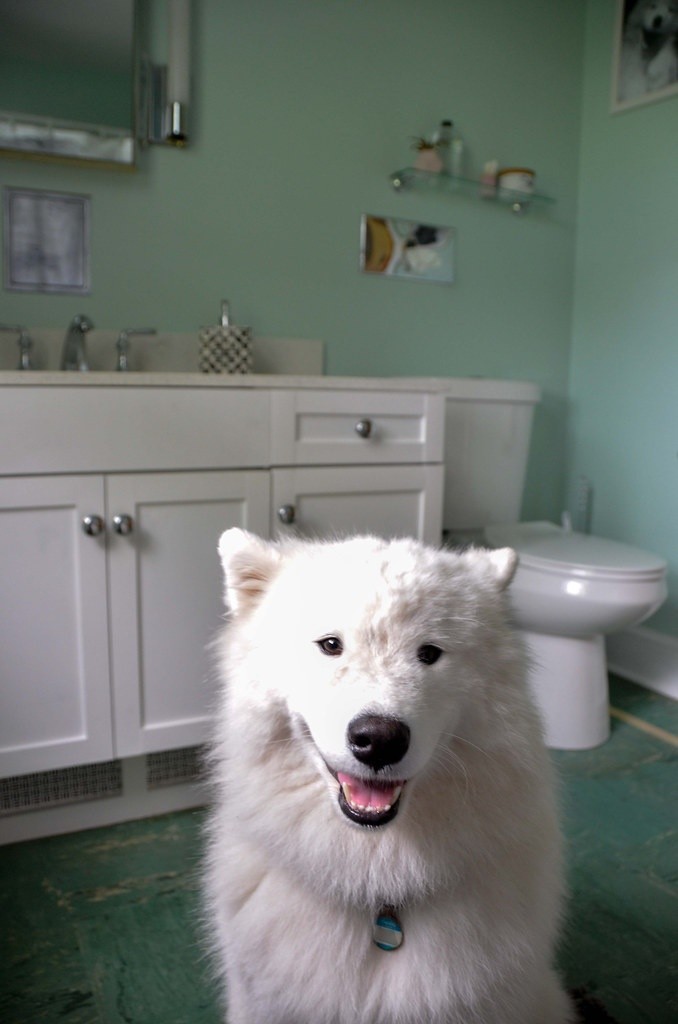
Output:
top-left (199, 323), bottom-right (250, 375)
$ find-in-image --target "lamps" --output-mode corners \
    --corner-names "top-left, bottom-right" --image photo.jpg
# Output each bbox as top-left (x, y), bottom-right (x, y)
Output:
top-left (160, 0), bottom-right (194, 146)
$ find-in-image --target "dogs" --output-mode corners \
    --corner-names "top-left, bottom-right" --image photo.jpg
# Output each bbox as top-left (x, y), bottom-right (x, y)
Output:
top-left (196, 526), bottom-right (580, 1024)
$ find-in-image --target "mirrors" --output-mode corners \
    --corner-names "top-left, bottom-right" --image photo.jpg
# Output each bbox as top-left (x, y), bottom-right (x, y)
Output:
top-left (0, 0), bottom-right (156, 136)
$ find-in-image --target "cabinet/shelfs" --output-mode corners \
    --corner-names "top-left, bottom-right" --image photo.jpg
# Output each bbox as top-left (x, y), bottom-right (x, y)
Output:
top-left (0, 384), bottom-right (451, 854)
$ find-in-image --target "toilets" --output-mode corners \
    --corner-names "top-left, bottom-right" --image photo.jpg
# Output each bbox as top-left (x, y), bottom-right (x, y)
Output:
top-left (405, 374), bottom-right (672, 750)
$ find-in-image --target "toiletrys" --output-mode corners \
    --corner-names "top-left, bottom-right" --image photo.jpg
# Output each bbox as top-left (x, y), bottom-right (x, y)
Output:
top-left (480, 158), bottom-right (537, 203)
top-left (197, 300), bottom-right (255, 375)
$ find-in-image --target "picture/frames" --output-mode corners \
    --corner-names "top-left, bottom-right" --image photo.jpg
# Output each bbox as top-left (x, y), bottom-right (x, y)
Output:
top-left (606, 0), bottom-right (678, 116)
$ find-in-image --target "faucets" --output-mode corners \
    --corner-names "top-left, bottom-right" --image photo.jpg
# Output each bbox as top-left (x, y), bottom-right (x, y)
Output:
top-left (59, 313), bottom-right (93, 371)
